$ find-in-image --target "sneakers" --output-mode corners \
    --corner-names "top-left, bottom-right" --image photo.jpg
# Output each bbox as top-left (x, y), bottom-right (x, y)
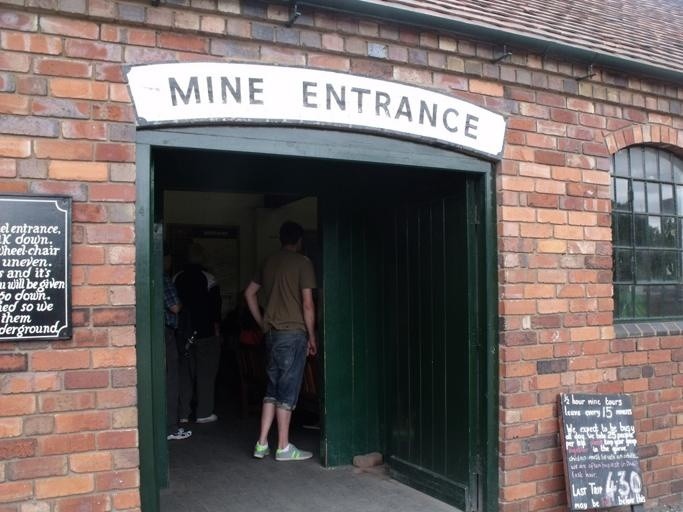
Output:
top-left (254, 441), bottom-right (270, 458)
top-left (167, 428), bottom-right (192, 440)
top-left (275, 443), bottom-right (313, 461)
top-left (180, 415), bottom-right (217, 424)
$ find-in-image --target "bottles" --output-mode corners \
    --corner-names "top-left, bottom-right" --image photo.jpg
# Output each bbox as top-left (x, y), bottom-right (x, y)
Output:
top-left (184, 330), bottom-right (197, 351)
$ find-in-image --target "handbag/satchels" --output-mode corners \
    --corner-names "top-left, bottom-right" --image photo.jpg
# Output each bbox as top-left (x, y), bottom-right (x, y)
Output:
top-left (177, 324), bottom-right (199, 359)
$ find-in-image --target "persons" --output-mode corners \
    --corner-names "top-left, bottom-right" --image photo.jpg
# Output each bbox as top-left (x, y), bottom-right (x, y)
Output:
top-left (162, 244), bottom-right (192, 441)
top-left (243, 218), bottom-right (318, 462)
top-left (171, 241), bottom-right (223, 424)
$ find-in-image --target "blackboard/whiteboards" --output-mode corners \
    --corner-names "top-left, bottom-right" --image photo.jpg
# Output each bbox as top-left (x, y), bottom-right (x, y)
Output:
top-left (0, 192), bottom-right (73, 341)
top-left (557, 392), bottom-right (646, 510)
